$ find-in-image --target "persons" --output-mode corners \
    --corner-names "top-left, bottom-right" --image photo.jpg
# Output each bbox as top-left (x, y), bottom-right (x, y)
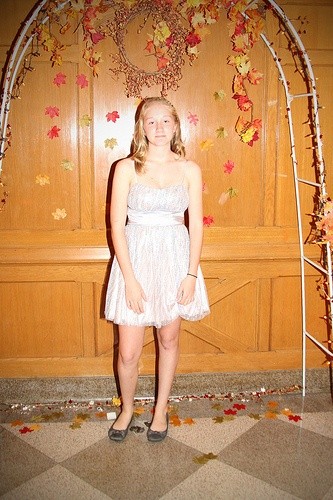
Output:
top-left (104, 97), bottom-right (210, 441)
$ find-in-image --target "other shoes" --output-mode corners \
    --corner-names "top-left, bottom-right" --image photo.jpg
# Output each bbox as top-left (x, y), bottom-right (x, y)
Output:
top-left (108, 411), bottom-right (134, 441)
top-left (147, 411), bottom-right (169, 442)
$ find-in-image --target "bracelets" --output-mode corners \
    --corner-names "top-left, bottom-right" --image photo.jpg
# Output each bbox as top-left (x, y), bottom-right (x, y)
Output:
top-left (187, 274), bottom-right (197, 278)
